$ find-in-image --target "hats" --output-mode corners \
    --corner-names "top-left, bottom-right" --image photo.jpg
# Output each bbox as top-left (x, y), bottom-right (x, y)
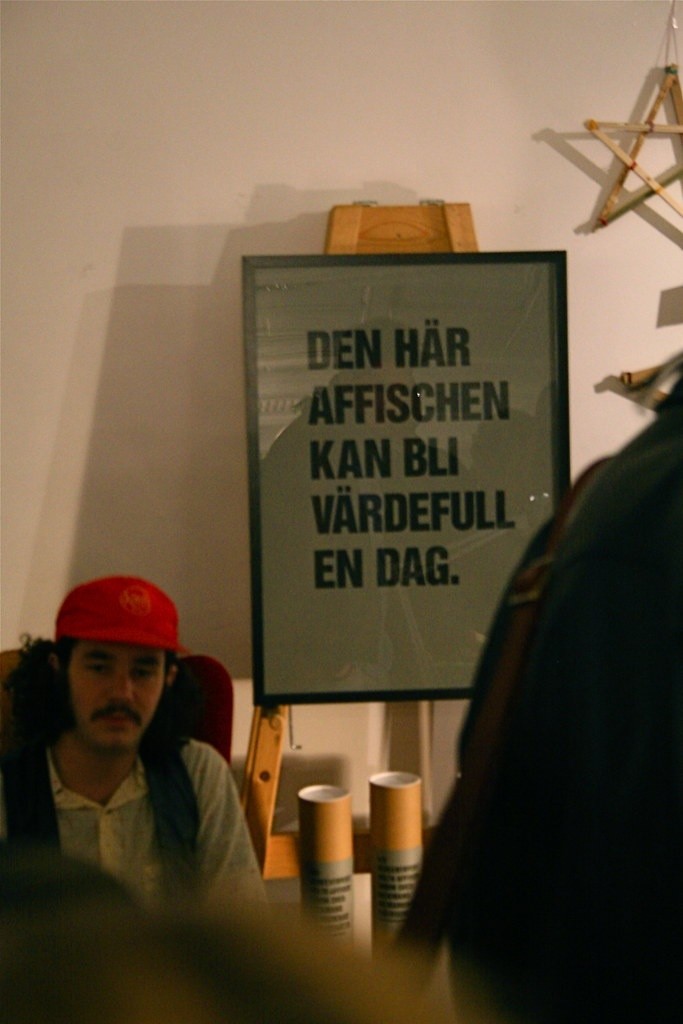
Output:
top-left (55, 575), bottom-right (186, 652)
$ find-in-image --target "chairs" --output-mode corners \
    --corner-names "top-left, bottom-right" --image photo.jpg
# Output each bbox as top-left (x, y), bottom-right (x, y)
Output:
top-left (1, 647), bottom-right (233, 761)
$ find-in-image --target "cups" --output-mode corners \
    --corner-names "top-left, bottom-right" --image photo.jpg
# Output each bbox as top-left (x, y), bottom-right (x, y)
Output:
top-left (368, 771), bottom-right (421, 965)
top-left (297, 785), bottom-right (355, 951)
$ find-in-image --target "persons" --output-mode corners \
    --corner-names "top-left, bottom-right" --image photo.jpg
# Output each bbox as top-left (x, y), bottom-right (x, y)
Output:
top-left (443, 373), bottom-right (683, 1024)
top-left (0, 575), bottom-right (277, 1024)
